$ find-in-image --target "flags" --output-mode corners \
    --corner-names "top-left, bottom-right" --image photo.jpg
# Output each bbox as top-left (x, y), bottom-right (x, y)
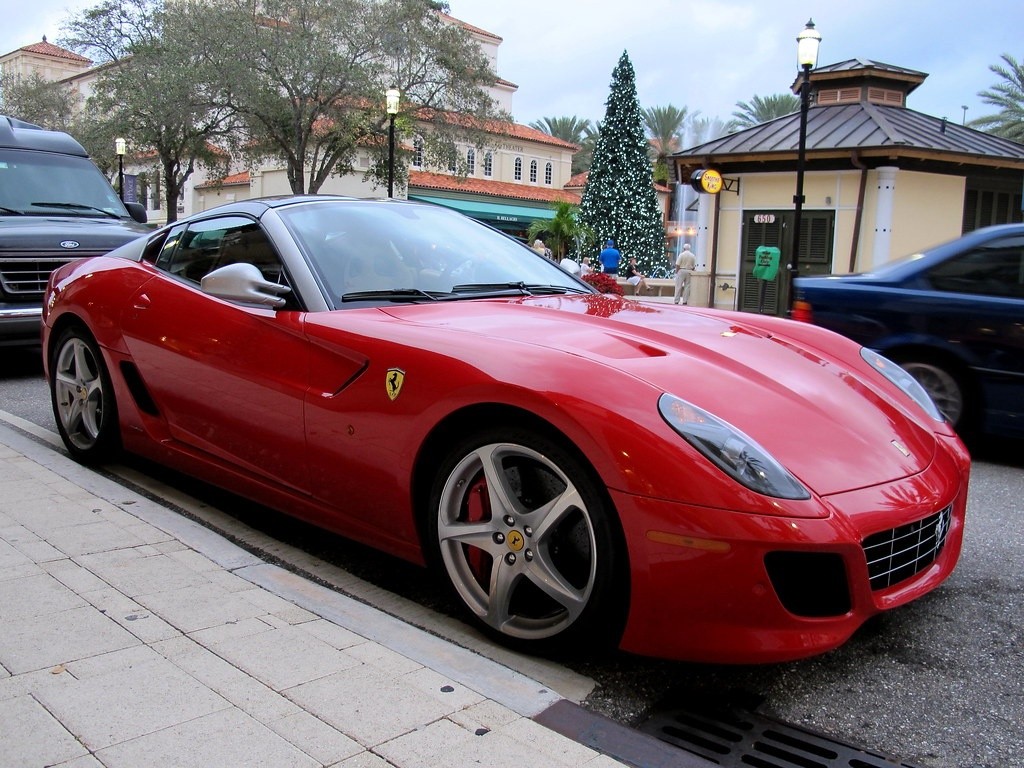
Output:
top-left (125, 176), bottom-right (138, 202)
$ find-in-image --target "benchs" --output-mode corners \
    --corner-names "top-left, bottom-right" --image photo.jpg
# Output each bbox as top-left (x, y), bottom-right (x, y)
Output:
top-left (616, 277), bottom-right (684, 296)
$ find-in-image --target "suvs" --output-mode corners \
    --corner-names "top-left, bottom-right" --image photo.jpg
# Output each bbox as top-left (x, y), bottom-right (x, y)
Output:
top-left (0, 115), bottom-right (167, 347)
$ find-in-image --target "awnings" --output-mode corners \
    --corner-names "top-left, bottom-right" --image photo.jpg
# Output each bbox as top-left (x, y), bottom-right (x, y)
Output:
top-left (407, 193), bottom-right (579, 231)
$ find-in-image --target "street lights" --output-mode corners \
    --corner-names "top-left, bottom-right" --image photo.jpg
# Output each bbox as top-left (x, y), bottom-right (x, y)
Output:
top-left (115, 138), bottom-right (128, 205)
top-left (385, 83), bottom-right (403, 195)
top-left (782, 16), bottom-right (828, 321)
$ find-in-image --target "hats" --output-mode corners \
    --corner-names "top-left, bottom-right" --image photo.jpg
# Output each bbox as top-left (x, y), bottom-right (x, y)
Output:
top-left (607, 240), bottom-right (614, 246)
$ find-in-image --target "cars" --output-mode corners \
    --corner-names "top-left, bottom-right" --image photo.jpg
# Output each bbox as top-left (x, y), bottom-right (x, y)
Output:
top-left (790, 221), bottom-right (1024, 438)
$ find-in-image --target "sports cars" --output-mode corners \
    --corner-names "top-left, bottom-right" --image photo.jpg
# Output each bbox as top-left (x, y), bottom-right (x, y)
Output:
top-left (40, 191), bottom-right (974, 672)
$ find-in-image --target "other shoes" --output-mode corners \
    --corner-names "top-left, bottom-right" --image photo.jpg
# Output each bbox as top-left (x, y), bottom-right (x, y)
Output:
top-left (683, 303), bottom-right (687, 305)
top-left (635, 292), bottom-right (640, 296)
top-left (646, 287), bottom-right (654, 291)
top-left (675, 302), bottom-right (678, 304)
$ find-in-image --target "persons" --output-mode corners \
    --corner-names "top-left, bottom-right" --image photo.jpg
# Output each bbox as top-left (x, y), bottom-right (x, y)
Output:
top-left (531, 239), bottom-right (654, 296)
top-left (674, 244), bottom-right (696, 306)
